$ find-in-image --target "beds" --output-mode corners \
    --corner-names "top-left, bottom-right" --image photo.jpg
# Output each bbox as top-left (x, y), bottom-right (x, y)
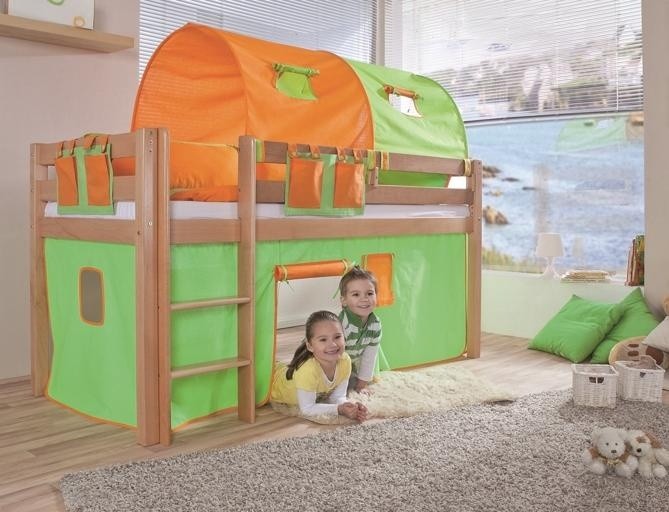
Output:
top-left (27, 126), bottom-right (484, 449)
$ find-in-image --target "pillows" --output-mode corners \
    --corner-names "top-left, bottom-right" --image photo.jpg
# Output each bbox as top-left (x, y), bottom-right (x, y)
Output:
top-left (643, 314), bottom-right (668, 356)
top-left (525, 292), bottom-right (629, 366)
top-left (589, 285), bottom-right (660, 365)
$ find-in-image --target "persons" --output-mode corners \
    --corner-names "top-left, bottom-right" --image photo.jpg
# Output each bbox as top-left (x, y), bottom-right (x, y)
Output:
top-left (337, 266), bottom-right (382, 399)
top-left (270, 310), bottom-right (368, 424)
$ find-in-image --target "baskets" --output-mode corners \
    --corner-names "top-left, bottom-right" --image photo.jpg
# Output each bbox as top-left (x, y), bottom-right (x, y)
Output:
top-left (616, 360), bottom-right (666, 403)
top-left (572, 363), bottom-right (618, 409)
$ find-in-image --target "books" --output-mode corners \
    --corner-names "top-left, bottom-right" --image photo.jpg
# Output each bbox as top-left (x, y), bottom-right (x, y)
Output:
top-left (560, 234), bottom-right (644, 287)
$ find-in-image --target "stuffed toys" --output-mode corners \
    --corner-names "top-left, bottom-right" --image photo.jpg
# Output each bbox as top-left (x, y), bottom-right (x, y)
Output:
top-left (625, 430), bottom-right (668, 479)
top-left (629, 356), bottom-right (657, 370)
top-left (583, 427), bottom-right (639, 478)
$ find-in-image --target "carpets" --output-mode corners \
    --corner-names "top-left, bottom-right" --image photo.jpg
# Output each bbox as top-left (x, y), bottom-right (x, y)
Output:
top-left (50, 376), bottom-right (668, 510)
top-left (268, 365), bottom-right (519, 425)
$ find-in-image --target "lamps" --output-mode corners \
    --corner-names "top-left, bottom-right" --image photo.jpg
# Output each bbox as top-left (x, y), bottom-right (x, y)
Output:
top-left (534, 229), bottom-right (566, 281)
